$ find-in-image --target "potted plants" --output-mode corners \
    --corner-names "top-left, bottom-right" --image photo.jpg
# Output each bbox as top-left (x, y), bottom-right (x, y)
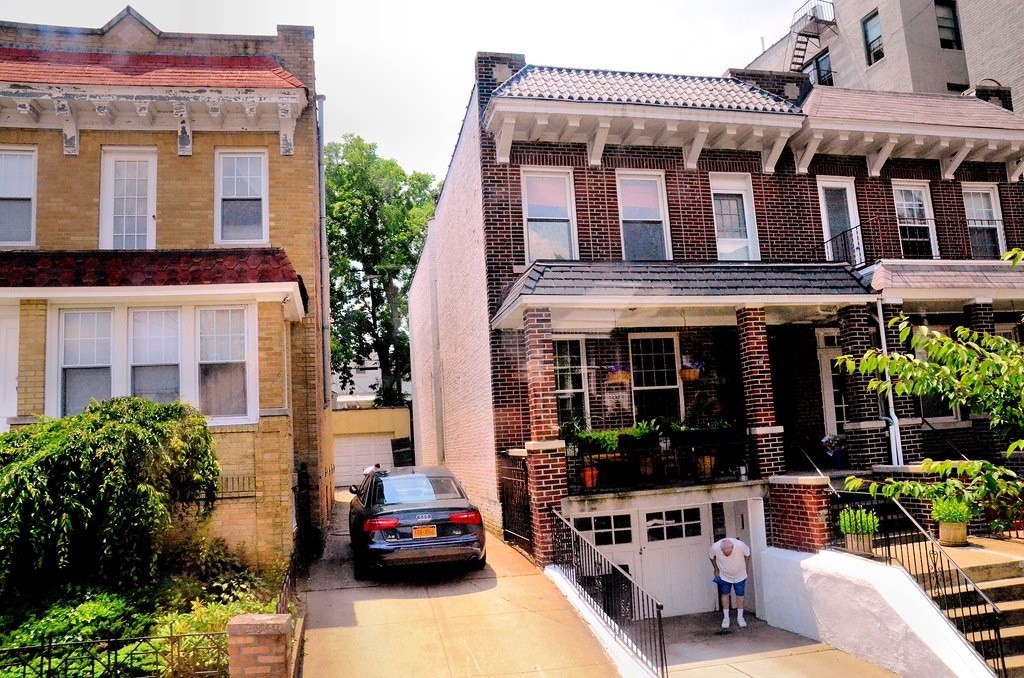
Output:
top-left (616, 419), bottom-right (664, 476)
top-left (575, 427), bottom-right (618, 489)
top-left (931, 492), bottom-right (980, 546)
top-left (838, 505), bottom-right (879, 552)
top-left (671, 391), bottom-right (731, 477)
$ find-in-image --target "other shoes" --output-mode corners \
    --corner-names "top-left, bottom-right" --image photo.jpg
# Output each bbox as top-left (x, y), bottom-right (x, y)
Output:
top-left (737, 617), bottom-right (748, 627)
top-left (721, 618), bottom-right (730, 628)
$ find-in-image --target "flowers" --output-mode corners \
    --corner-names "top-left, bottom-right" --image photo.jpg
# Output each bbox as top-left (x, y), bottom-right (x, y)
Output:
top-left (681, 362), bottom-right (705, 372)
top-left (607, 365), bottom-right (630, 372)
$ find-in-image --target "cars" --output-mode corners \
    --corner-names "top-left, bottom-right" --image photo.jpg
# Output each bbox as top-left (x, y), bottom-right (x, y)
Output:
top-left (349, 465), bottom-right (486, 580)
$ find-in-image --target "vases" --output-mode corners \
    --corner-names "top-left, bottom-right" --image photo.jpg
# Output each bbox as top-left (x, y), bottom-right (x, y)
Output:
top-left (679, 368), bottom-right (700, 382)
top-left (607, 371), bottom-right (631, 386)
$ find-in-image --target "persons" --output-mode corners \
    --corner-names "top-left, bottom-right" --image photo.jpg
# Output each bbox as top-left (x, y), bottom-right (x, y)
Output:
top-left (709, 538), bottom-right (750, 628)
top-left (362, 463), bottom-right (380, 476)
